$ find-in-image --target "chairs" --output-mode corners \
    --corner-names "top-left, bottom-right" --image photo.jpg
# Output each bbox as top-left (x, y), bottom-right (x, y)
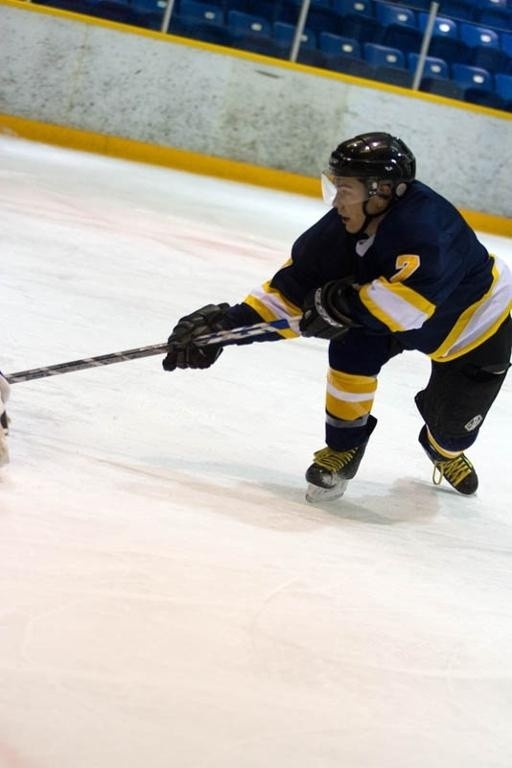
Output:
top-left (32, 1), bottom-right (511, 116)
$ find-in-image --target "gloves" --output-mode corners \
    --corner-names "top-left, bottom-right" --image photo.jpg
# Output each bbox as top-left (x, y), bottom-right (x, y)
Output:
top-left (299, 282), bottom-right (361, 341)
top-left (162, 302), bottom-right (245, 372)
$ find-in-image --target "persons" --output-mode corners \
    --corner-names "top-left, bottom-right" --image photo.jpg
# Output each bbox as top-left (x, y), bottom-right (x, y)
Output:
top-left (166, 131), bottom-right (512, 502)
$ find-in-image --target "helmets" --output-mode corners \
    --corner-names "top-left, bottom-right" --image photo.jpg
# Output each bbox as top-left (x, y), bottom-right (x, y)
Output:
top-left (320, 131), bottom-right (417, 204)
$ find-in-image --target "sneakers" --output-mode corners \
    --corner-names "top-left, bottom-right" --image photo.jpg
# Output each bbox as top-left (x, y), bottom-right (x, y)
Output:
top-left (420, 427), bottom-right (479, 495)
top-left (306, 443), bottom-right (367, 488)
